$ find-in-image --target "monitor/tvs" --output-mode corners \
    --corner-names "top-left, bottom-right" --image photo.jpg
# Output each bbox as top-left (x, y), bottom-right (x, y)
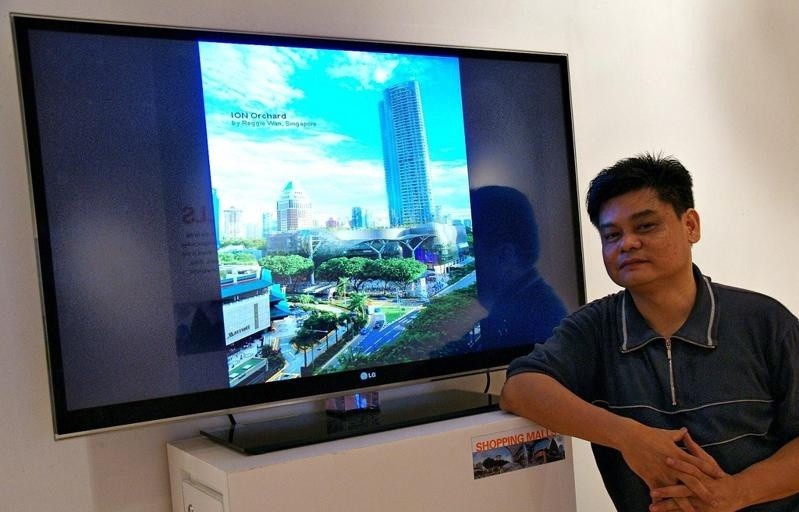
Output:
top-left (8, 10), bottom-right (586, 455)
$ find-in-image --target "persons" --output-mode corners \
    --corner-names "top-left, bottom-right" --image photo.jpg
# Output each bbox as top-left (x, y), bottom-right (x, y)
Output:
top-left (499, 155), bottom-right (799, 512)
top-left (428, 186), bottom-right (568, 358)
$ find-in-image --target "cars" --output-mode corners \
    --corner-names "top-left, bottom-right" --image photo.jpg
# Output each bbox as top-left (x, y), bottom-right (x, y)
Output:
top-left (375, 307), bottom-right (380, 312)
top-left (374, 323), bottom-right (380, 328)
top-left (348, 312), bottom-right (356, 321)
top-left (361, 329), bottom-right (367, 334)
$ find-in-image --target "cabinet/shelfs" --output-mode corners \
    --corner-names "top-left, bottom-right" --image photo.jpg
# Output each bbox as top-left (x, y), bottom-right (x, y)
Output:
top-left (165, 411), bottom-right (579, 511)
